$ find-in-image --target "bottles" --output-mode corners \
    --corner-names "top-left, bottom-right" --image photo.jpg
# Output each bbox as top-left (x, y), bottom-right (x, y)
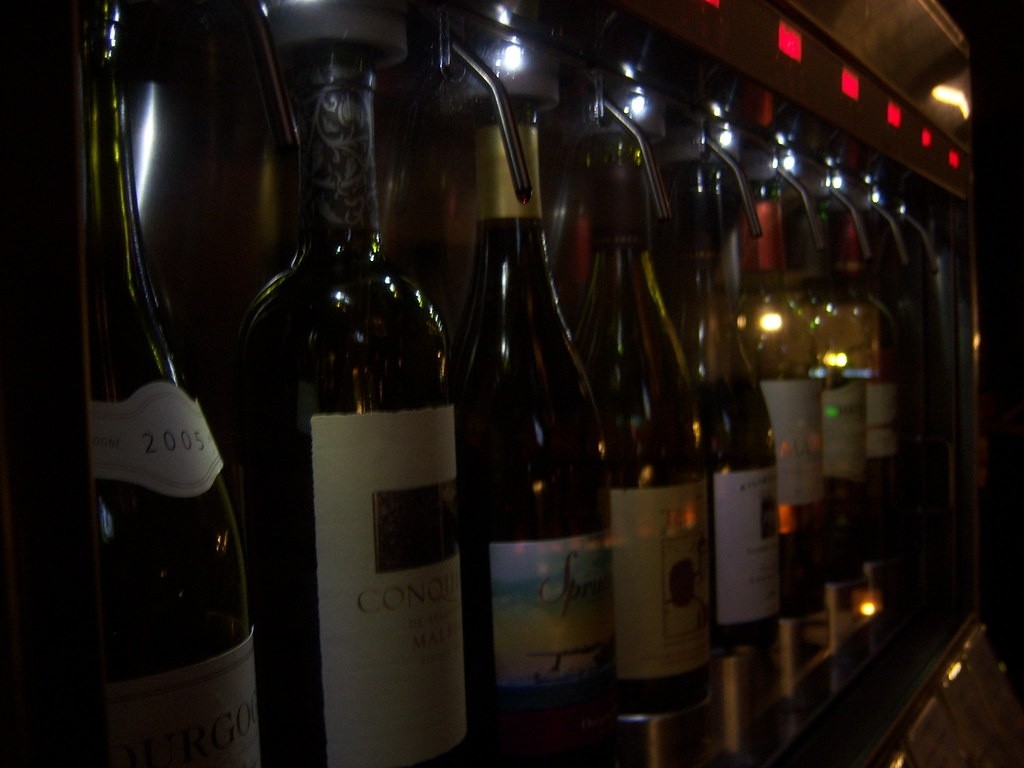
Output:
top-left (57, 0), bottom-right (948, 768)
